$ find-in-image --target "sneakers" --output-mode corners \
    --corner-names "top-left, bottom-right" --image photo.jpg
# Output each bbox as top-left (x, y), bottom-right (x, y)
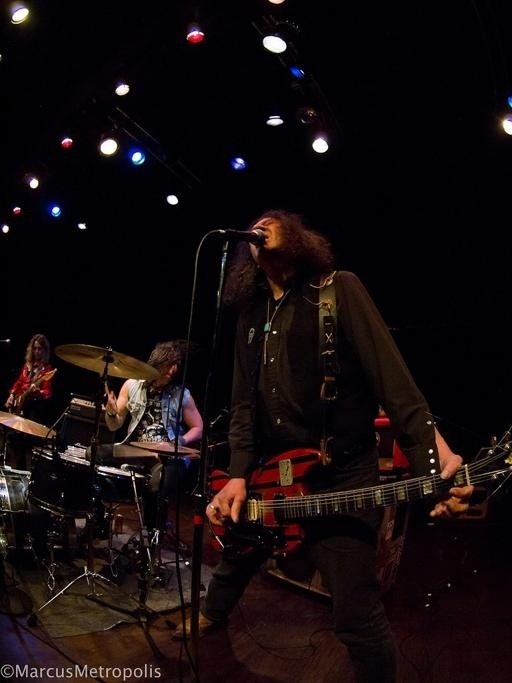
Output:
top-left (75, 517), bottom-right (112, 544)
top-left (170, 611), bottom-right (217, 641)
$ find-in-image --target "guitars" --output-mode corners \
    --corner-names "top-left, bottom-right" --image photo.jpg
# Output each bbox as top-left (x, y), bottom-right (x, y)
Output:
top-left (208, 435), bottom-right (511, 556)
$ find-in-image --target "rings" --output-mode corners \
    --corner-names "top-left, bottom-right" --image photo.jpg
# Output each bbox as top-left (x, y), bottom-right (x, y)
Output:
top-left (208, 504), bottom-right (216, 510)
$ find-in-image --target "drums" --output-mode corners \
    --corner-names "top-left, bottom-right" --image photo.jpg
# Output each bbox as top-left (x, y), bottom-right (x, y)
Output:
top-left (24, 447), bottom-right (93, 516)
top-left (94, 464), bottom-right (144, 505)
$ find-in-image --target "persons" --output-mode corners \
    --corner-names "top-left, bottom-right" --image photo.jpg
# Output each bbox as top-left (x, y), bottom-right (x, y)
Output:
top-left (5, 334), bottom-right (58, 471)
top-left (73, 339), bottom-right (203, 584)
top-left (173, 208), bottom-right (473, 682)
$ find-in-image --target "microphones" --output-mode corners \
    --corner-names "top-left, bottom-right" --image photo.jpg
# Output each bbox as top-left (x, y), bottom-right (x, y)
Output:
top-left (225, 229), bottom-right (264, 243)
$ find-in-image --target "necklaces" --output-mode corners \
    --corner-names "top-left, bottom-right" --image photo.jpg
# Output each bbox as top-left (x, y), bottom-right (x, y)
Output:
top-left (262, 287), bottom-right (292, 363)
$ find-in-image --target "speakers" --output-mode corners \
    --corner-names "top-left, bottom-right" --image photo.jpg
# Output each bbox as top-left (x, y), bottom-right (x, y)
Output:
top-left (60, 414), bottom-right (115, 452)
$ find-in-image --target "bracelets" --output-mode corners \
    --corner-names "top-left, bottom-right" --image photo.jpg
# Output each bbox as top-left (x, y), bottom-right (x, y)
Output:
top-left (106, 411), bottom-right (118, 418)
top-left (10, 393), bottom-right (15, 397)
top-left (173, 435), bottom-right (187, 445)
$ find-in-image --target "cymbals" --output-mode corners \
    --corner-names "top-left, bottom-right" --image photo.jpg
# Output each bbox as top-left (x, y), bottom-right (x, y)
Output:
top-left (0, 410), bottom-right (56, 440)
top-left (130, 440), bottom-right (198, 455)
top-left (55, 345), bottom-right (160, 381)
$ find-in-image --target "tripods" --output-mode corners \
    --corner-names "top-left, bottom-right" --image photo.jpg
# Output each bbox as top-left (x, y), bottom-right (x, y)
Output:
top-left (84, 471), bottom-right (190, 661)
top-left (26, 464), bottom-right (178, 631)
